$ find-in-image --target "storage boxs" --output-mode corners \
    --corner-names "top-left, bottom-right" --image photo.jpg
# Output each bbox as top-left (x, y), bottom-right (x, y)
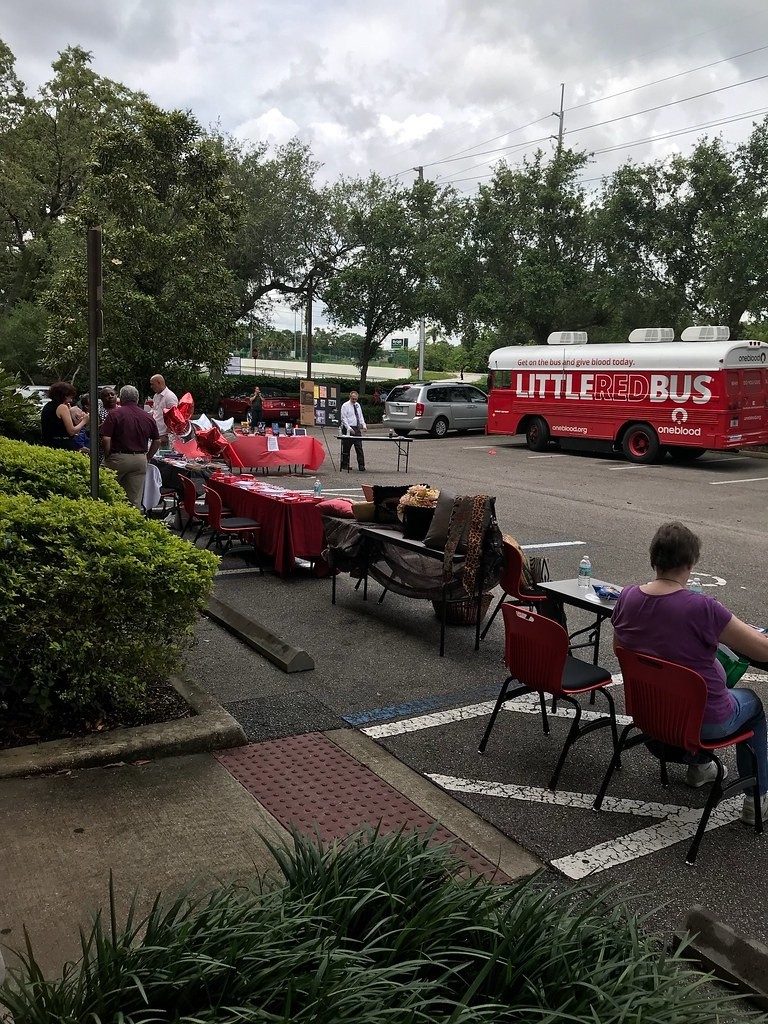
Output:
top-left (175, 507), bottom-right (199, 531)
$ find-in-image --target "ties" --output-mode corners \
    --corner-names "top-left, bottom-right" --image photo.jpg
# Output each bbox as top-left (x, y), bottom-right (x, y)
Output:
top-left (353, 405), bottom-right (360, 433)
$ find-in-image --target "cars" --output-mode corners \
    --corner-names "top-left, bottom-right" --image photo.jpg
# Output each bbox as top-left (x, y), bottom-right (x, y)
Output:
top-left (13, 386), bottom-right (52, 413)
top-left (217, 385), bottom-right (300, 426)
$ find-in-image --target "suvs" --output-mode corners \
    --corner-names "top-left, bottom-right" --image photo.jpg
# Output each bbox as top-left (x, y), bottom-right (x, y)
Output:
top-left (382, 380), bottom-right (489, 440)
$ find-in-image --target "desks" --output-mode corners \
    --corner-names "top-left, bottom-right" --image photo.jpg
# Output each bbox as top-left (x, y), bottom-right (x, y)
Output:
top-left (322, 515), bottom-right (482, 659)
top-left (225, 434), bottom-right (315, 477)
top-left (154, 456), bottom-right (230, 505)
top-left (209, 475), bottom-right (338, 582)
top-left (334, 434), bottom-right (414, 474)
top-left (532, 575), bottom-right (768, 714)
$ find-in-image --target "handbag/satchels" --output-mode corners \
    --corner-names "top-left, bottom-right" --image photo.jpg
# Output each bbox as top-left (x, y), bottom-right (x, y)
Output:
top-left (716, 646), bottom-right (750, 690)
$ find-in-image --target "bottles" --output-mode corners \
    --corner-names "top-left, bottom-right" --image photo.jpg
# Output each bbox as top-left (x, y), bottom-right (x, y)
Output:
top-left (347, 427), bottom-right (350, 436)
top-left (578, 556), bottom-right (591, 588)
top-left (338, 426), bottom-right (342, 436)
top-left (314, 480), bottom-right (321, 498)
top-left (690, 578), bottom-right (702, 595)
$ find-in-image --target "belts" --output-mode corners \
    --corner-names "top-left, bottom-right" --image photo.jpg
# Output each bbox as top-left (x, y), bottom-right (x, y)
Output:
top-left (110, 450), bottom-right (144, 454)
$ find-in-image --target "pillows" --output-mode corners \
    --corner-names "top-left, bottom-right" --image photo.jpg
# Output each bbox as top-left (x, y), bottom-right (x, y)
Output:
top-left (420, 487), bottom-right (496, 553)
top-left (395, 485), bottom-right (440, 522)
top-left (371, 482), bottom-right (438, 522)
top-left (352, 500), bottom-right (374, 521)
top-left (402, 504), bottom-right (434, 539)
top-left (362, 484), bottom-right (374, 501)
top-left (502, 533), bottom-right (533, 590)
top-left (481, 496), bottom-right (503, 551)
top-left (313, 497), bottom-right (356, 519)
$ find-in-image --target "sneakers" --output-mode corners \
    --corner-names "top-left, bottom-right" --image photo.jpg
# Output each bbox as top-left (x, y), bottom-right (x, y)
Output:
top-left (743, 791), bottom-right (768, 824)
top-left (685, 764), bottom-right (728, 787)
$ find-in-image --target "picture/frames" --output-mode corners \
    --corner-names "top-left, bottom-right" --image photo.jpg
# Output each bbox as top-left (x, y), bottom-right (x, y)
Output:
top-left (316, 410), bottom-right (325, 424)
top-left (320, 386), bottom-right (327, 398)
top-left (320, 399), bottom-right (325, 407)
top-left (329, 400), bottom-right (337, 407)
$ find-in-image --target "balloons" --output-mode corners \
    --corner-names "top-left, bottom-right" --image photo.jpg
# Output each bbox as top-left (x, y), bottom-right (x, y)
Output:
top-left (163, 392), bottom-right (238, 457)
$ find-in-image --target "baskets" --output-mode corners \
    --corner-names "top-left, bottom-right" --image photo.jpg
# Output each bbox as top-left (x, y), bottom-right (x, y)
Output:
top-left (432, 592), bottom-right (494, 625)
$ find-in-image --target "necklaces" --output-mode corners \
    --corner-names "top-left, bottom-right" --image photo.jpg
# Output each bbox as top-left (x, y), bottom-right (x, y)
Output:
top-left (655, 578), bottom-right (683, 588)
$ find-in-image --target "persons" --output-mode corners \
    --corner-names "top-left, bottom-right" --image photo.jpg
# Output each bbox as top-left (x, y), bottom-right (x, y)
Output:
top-left (250, 386), bottom-right (265, 427)
top-left (41, 374), bottom-right (178, 511)
top-left (611, 521), bottom-right (768, 825)
top-left (341, 391), bottom-right (367, 471)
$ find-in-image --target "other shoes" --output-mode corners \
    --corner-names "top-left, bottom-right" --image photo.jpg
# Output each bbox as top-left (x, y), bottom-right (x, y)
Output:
top-left (343, 466), bottom-right (352, 470)
top-left (359, 465), bottom-right (364, 471)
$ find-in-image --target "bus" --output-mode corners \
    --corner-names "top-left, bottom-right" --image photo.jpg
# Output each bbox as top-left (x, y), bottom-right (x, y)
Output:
top-left (484, 325), bottom-right (768, 464)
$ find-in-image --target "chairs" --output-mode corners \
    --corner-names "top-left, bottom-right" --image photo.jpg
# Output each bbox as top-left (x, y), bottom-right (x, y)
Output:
top-left (480, 537), bottom-right (548, 639)
top-left (589, 646), bottom-right (766, 866)
top-left (476, 602), bottom-right (623, 791)
top-left (202, 484), bottom-right (265, 576)
top-left (145, 463), bottom-right (176, 518)
top-left (173, 472), bottom-right (233, 547)
